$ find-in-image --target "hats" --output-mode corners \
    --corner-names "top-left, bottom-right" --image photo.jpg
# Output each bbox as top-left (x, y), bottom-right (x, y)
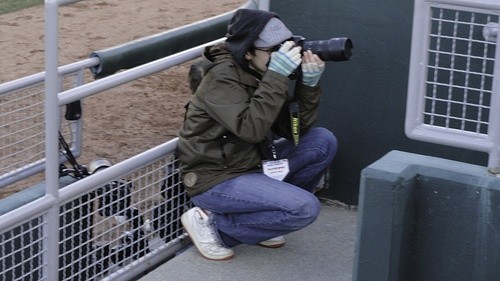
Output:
top-left (254, 17), bottom-right (293, 49)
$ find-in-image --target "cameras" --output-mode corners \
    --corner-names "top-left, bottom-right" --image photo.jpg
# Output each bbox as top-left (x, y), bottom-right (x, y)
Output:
top-left (271, 36), bottom-right (353, 80)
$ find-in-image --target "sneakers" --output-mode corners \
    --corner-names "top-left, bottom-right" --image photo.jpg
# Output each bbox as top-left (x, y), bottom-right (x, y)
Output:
top-left (181, 206), bottom-right (234, 259)
top-left (260, 235), bottom-right (285, 248)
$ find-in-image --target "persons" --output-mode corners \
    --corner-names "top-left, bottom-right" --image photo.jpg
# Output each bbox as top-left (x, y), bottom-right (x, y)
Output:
top-left (176, 6), bottom-right (338, 260)
